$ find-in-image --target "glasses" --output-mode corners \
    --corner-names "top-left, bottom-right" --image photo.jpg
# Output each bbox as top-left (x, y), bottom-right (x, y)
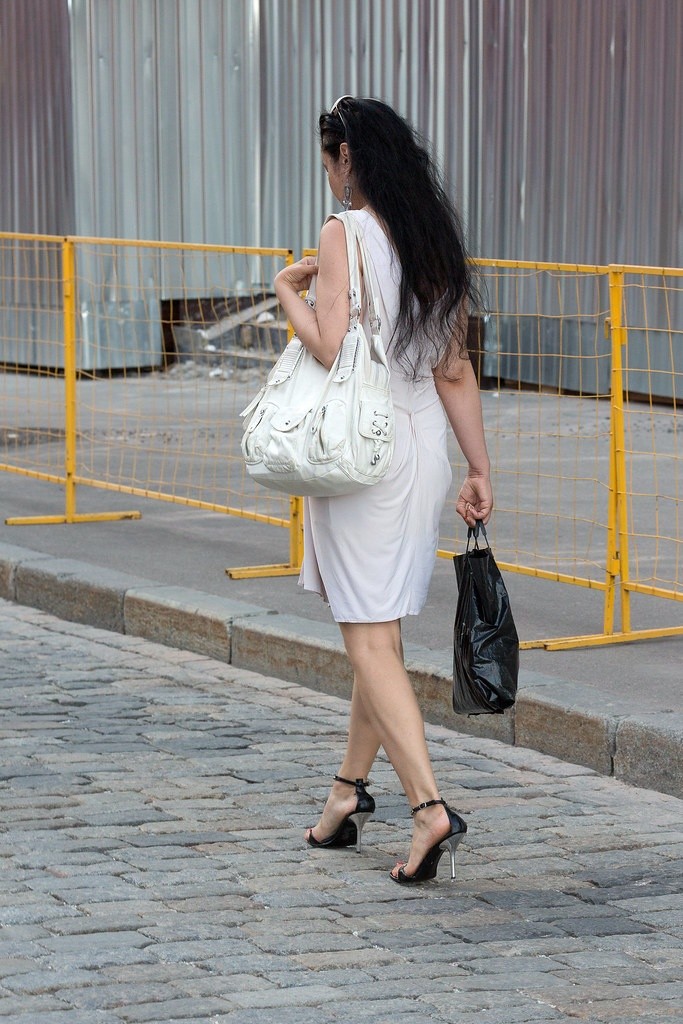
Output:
top-left (329, 95), bottom-right (382, 142)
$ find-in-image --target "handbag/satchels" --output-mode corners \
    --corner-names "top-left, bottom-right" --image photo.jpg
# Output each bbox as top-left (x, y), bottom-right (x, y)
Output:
top-left (238, 214), bottom-right (394, 498)
top-left (452, 518), bottom-right (520, 717)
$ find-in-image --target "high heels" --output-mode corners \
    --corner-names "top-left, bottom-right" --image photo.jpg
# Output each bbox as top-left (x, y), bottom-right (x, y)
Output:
top-left (306, 775), bottom-right (376, 853)
top-left (388, 796), bottom-right (467, 886)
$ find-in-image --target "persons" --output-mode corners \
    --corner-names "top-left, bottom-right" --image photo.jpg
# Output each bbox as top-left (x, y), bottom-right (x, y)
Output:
top-left (273, 96), bottom-right (492, 883)
